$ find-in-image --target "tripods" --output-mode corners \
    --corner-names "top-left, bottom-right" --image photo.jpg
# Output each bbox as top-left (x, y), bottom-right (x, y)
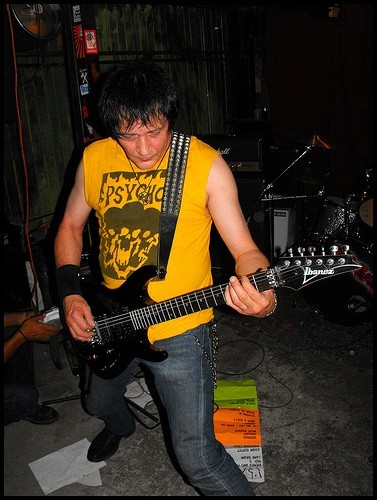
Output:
top-left (321, 199), bottom-right (369, 249)
top-left (41, 394), bottom-right (160, 423)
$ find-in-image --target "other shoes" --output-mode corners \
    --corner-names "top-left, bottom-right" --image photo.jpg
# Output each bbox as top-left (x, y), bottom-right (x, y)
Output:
top-left (19, 404), bottom-right (59, 425)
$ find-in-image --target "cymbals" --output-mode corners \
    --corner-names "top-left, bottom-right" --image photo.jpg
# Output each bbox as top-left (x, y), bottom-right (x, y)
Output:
top-left (359, 198), bottom-right (373, 227)
top-left (289, 171), bottom-right (333, 186)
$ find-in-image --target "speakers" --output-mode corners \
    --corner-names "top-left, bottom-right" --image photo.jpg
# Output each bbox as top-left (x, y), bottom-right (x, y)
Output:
top-left (207, 179), bottom-right (269, 269)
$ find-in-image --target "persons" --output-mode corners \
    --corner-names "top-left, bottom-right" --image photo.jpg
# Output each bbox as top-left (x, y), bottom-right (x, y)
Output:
top-left (52, 62), bottom-right (277, 496)
top-left (4, 311), bottom-right (63, 425)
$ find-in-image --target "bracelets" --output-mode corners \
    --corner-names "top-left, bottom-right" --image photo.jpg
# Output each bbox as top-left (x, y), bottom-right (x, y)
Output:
top-left (267, 291), bottom-right (277, 316)
top-left (17, 328), bottom-right (28, 343)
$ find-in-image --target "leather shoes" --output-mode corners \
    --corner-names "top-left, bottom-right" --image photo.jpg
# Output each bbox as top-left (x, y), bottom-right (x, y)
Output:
top-left (87, 421), bottom-right (136, 462)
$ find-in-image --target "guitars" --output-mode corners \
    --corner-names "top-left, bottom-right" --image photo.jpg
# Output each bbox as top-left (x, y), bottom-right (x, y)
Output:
top-left (59, 243), bottom-right (363, 380)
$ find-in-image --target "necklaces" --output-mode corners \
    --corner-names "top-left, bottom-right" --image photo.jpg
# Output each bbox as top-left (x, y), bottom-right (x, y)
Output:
top-left (122, 135), bottom-right (172, 205)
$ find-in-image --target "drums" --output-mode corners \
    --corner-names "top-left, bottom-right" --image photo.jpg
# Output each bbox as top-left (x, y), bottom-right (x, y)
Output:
top-left (302, 221), bottom-right (375, 327)
top-left (317, 196), bottom-right (359, 240)
